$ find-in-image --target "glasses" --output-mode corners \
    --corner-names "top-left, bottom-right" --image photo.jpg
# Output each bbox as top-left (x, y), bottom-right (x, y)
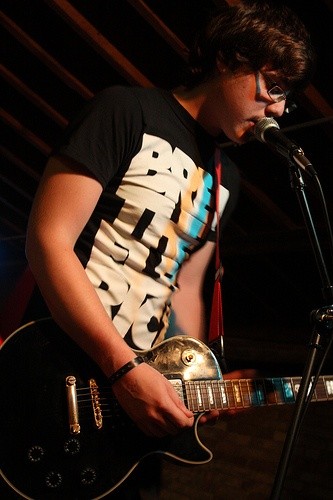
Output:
top-left (247, 58), bottom-right (298, 114)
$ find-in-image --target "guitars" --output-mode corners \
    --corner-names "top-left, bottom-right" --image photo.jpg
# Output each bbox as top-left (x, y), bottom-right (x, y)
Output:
top-left (0, 315), bottom-right (333, 500)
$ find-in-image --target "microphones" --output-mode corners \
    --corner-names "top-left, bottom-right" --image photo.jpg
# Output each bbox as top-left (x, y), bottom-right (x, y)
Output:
top-left (254, 117), bottom-right (317, 177)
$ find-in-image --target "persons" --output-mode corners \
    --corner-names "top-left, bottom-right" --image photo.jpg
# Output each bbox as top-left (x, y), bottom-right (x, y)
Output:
top-left (16, 0), bottom-right (316, 500)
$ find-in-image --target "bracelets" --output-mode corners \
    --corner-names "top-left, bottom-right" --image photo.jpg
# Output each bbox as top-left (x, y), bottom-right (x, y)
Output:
top-left (106, 356), bottom-right (143, 386)
